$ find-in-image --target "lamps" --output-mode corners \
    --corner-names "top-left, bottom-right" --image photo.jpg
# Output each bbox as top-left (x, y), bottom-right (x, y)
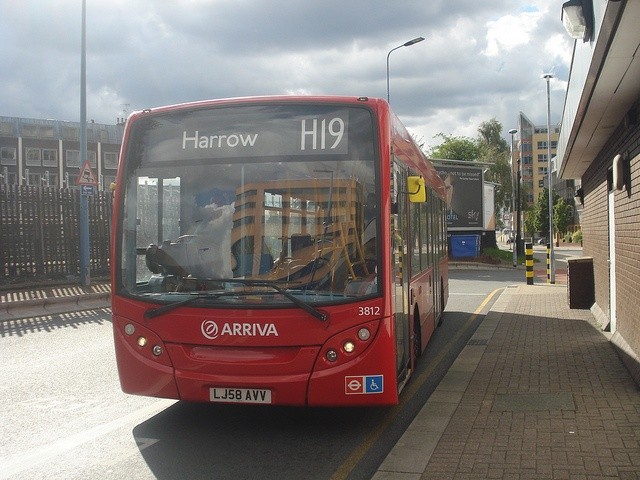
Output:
top-left (561, 1), bottom-right (592, 44)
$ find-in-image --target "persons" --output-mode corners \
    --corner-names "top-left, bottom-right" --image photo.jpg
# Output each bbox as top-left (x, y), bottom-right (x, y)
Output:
top-left (363, 193), bottom-right (376, 222)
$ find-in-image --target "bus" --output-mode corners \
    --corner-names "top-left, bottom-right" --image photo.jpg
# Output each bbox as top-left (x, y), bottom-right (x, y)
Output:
top-left (109, 95), bottom-right (449, 409)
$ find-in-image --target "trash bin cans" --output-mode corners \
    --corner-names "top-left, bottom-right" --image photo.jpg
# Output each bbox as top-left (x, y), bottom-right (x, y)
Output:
top-left (567, 257), bottom-right (594, 309)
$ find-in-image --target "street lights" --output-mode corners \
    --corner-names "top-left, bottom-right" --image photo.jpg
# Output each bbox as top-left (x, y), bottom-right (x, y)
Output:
top-left (544, 75), bottom-right (554, 283)
top-left (509, 130), bottom-right (517, 266)
top-left (387, 37), bottom-right (424, 103)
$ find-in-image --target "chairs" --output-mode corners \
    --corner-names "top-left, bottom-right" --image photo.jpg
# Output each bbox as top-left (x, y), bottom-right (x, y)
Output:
top-left (233, 252), bottom-right (271, 277)
